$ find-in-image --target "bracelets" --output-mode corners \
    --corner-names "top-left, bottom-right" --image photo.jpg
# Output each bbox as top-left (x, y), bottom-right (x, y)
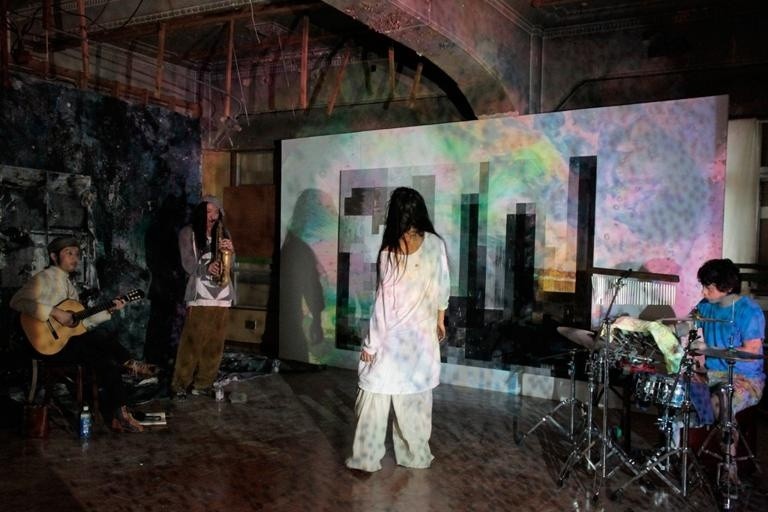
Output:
top-left (106, 307), bottom-right (116, 314)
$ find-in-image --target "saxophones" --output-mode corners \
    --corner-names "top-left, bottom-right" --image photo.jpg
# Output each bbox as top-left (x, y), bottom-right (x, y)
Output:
top-left (210, 218), bottom-right (232, 287)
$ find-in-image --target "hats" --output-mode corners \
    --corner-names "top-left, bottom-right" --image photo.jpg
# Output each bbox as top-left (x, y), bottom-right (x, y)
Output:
top-left (195, 195), bottom-right (226, 218)
top-left (47, 236), bottom-right (81, 252)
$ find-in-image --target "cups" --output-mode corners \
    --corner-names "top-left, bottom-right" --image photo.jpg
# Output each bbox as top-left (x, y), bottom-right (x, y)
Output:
top-left (272, 359), bottom-right (281, 373)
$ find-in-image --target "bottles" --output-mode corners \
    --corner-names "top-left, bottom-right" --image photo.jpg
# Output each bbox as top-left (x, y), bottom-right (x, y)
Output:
top-left (80, 406), bottom-right (91, 439)
top-left (215, 384), bottom-right (225, 400)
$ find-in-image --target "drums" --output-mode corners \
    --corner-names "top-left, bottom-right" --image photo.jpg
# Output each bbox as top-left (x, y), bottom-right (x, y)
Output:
top-left (635, 373), bottom-right (693, 410)
top-left (612, 343), bottom-right (659, 374)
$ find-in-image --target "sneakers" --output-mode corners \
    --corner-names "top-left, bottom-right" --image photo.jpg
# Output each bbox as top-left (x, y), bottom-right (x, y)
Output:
top-left (172, 387), bottom-right (215, 402)
top-left (119, 357), bottom-right (161, 383)
top-left (107, 403), bottom-right (145, 433)
top-left (346, 455), bottom-right (382, 473)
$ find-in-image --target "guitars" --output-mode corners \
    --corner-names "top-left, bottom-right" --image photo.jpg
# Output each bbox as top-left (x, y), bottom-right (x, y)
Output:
top-left (20, 288), bottom-right (144, 355)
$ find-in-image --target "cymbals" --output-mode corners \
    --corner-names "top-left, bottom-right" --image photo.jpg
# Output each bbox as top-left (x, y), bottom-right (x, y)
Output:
top-left (580, 266), bottom-right (677, 283)
top-left (558, 325), bottom-right (607, 351)
top-left (696, 348), bottom-right (762, 360)
top-left (656, 317), bottom-right (731, 326)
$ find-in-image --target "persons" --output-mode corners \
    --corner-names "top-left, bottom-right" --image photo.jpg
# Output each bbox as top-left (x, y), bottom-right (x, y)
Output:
top-left (640, 256), bottom-right (768, 501)
top-left (341, 187), bottom-right (452, 474)
top-left (168, 193), bottom-right (242, 402)
top-left (6, 238), bottom-right (163, 433)
